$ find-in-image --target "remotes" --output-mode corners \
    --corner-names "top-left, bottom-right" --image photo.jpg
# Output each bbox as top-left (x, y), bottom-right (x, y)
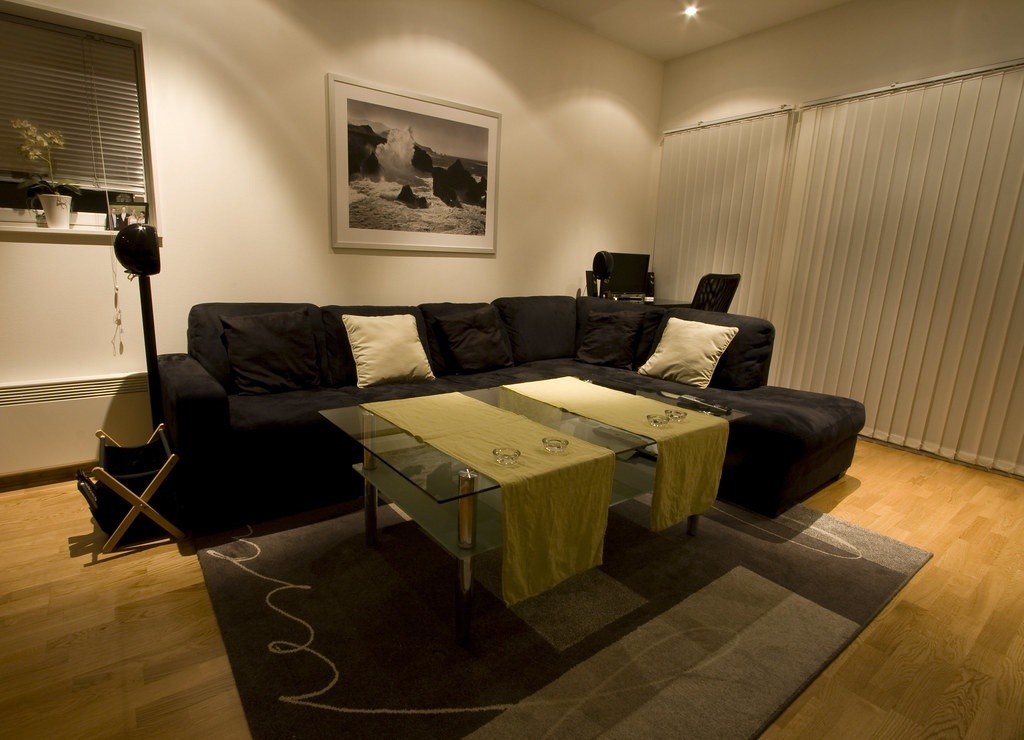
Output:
top-left (678, 394), bottom-right (732, 415)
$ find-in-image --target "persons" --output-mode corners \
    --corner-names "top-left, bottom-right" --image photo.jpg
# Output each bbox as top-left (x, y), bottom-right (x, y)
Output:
top-left (107, 208), bottom-right (119, 229)
top-left (117, 206), bottom-right (145, 228)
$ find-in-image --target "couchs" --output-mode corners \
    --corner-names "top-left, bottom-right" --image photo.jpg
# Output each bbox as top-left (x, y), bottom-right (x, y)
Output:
top-left (156, 295), bottom-right (867, 538)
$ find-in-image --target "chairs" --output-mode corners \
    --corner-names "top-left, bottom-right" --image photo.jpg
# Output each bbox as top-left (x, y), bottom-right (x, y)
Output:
top-left (691, 272), bottom-right (742, 313)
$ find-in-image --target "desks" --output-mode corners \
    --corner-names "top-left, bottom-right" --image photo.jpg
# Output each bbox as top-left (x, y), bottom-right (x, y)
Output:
top-left (619, 297), bottom-right (692, 307)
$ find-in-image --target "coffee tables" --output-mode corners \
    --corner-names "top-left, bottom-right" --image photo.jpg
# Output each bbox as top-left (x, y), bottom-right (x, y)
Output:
top-left (318, 369), bottom-right (752, 647)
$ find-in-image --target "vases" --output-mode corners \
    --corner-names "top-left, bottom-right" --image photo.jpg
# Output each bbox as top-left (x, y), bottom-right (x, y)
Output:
top-left (37, 193), bottom-right (72, 230)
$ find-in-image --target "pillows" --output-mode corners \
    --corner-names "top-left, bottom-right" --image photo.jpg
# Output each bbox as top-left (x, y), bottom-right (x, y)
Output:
top-left (573, 309), bottom-right (647, 371)
top-left (637, 316), bottom-right (740, 391)
top-left (434, 304), bottom-right (516, 374)
top-left (218, 306), bottom-right (325, 396)
top-left (341, 313), bottom-right (436, 389)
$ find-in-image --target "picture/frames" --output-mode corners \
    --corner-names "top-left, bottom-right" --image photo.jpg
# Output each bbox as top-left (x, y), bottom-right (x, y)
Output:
top-left (106, 202), bottom-right (149, 231)
top-left (324, 71), bottom-right (503, 255)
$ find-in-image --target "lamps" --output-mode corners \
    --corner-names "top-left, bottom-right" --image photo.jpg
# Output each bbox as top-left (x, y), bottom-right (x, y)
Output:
top-left (112, 223), bottom-right (162, 433)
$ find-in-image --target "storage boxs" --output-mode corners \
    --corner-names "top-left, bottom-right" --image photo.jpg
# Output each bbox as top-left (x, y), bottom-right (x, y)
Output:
top-left (91, 423), bottom-right (185, 555)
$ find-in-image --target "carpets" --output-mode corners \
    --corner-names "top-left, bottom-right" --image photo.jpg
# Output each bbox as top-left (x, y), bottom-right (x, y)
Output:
top-left (196, 457), bottom-right (934, 740)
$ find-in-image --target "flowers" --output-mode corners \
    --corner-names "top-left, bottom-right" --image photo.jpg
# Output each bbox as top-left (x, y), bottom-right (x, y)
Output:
top-left (11, 118), bottom-right (83, 219)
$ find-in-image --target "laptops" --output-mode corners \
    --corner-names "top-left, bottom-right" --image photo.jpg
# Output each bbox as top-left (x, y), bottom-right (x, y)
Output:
top-left (592, 252), bottom-right (650, 304)
